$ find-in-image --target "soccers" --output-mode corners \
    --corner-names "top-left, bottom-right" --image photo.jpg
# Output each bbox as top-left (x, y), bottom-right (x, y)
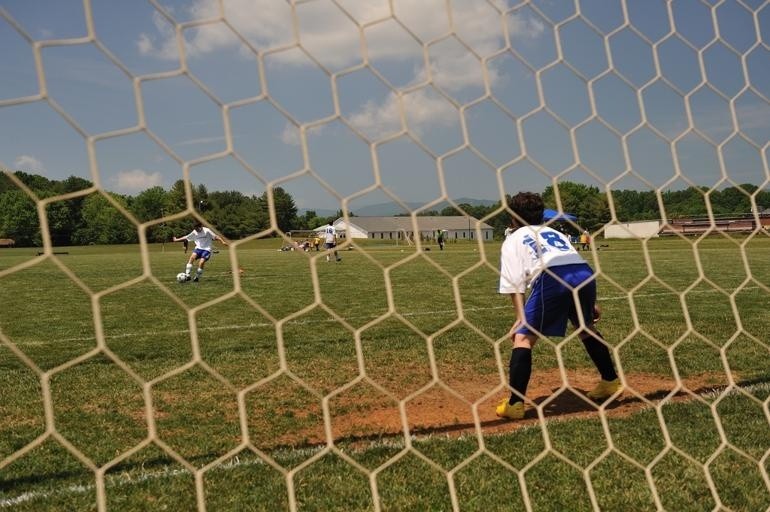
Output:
top-left (177, 272), bottom-right (187, 283)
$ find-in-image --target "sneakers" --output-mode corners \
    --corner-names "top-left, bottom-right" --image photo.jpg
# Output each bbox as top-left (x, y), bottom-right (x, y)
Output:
top-left (586, 379), bottom-right (624, 400)
top-left (185, 273), bottom-right (199, 282)
top-left (496, 398), bottom-right (526, 420)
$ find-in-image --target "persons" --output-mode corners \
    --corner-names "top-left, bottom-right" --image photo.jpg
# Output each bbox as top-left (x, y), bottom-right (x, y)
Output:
top-left (583, 226), bottom-right (589, 249)
top-left (172, 218), bottom-right (228, 282)
top-left (183, 239), bottom-right (188, 253)
top-left (496, 191), bottom-right (624, 419)
top-left (558, 222), bottom-right (564, 234)
top-left (286, 235), bottom-right (320, 252)
top-left (325, 219), bottom-right (341, 261)
top-left (565, 230), bottom-right (571, 244)
top-left (504, 223), bottom-right (517, 239)
top-left (437, 229), bottom-right (444, 250)
